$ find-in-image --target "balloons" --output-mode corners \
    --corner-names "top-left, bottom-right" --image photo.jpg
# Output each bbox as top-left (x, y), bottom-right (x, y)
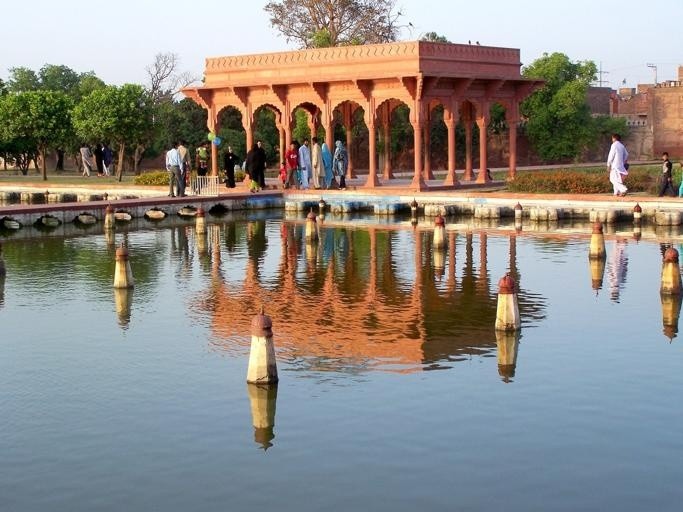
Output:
top-left (207, 133), bottom-right (216, 141)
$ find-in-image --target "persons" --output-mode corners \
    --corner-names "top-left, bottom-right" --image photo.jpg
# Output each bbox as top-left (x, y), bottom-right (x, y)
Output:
top-left (657, 153), bottom-right (676, 197)
top-left (134, 139), bottom-right (146, 176)
top-left (607, 133), bottom-right (629, 196)
top-left (165, 136), bottom-right (349, 198)
top-left (94, 144), bottom-right (104, 177)
top-left (102, 143), bottom-right (114, 176)
top-left (607, 239), bottom-right (628, 304)
top-left (171, 221), bottom-right (350, 277)
top-left (79, 143), bottom-right (92, 177)
